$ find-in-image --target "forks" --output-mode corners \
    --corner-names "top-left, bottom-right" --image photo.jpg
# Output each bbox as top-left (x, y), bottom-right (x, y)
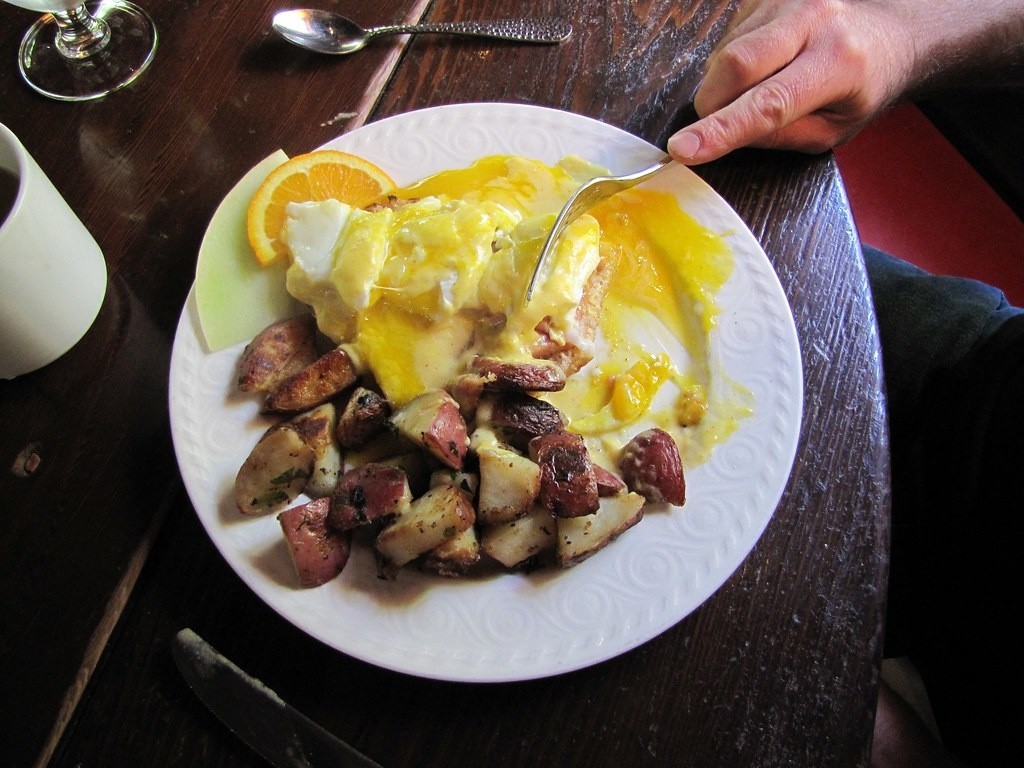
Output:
top-left (526, 159), bottom-right (673, 303)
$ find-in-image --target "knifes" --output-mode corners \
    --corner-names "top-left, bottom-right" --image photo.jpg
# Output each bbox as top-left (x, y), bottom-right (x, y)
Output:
top-left (178, 630), bottom-right (379, 768)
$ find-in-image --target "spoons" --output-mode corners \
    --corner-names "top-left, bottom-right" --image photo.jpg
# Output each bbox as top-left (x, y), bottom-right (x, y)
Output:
top-left (273, 8), bottom-right (574, 55)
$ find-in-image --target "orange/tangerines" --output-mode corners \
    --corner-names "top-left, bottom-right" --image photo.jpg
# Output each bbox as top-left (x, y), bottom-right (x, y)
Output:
top-left (248, 151), bottom-right (396, 266)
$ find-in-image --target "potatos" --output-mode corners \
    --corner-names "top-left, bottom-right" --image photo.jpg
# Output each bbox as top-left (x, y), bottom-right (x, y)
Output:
top-left (235, 315), bottom-right (686, 588)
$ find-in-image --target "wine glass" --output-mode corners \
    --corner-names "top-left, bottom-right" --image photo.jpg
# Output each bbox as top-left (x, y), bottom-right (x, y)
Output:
top-left (0, 0), bottom-right (158, 100)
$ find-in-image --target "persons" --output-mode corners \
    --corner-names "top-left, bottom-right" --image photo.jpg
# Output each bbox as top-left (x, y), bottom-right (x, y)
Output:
top-left (665, 0), bottom-right (1024, 768)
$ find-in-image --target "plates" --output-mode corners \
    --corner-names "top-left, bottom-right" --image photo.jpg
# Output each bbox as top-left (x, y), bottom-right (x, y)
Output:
top-left (167, 103), bottom-right (803, 685)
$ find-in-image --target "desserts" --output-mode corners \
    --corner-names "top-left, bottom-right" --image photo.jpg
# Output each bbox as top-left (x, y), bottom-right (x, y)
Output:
top-left (276, 192), bottom-right (613, 397)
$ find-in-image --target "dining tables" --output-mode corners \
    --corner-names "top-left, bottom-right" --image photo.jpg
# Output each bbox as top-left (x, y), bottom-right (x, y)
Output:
top-left (0, 0), bottom-right (893, 768)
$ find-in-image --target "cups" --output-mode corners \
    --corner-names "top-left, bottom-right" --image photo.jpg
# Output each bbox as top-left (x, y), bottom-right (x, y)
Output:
top-left (0, 122), bottom-right (108, 380)
